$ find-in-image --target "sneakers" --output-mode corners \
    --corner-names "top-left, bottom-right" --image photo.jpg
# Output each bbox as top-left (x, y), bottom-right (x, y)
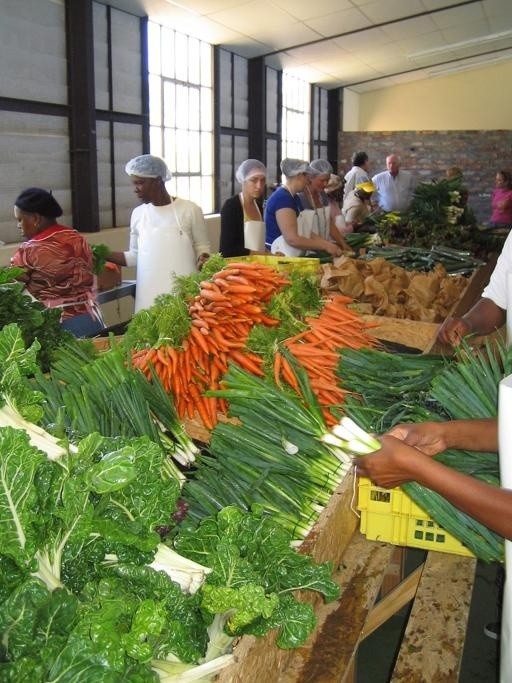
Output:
top-left (483, 621), bottom-right (500, 640)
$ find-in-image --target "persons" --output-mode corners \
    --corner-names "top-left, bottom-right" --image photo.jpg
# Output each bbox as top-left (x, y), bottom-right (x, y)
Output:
top-left (353, 373), bottom-right (512, 683)
top-left (10, 188), bottom-right (105, 340)
top-left (485, 171), bottom-right (512, 228)
top-left (439, 230), bottom-right (512, 639)
top-left (264, 150), bottom-right (422, 260)
top-left (446, 167), bottom-right (468, 205)
top-left (219, 158), bottom-right (285, 256)
top-left (106, 155), bottom-right (209, 317)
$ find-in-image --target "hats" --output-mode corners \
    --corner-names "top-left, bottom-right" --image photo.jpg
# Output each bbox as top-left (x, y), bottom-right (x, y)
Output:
top-left (14, 187), bottom-right (63, 218)
top-left (355, 175), bottom-right (377, 193)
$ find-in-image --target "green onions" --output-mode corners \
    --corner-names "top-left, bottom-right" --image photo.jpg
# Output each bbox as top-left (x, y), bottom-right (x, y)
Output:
top-left (30, 331), bottom-right (512, 566)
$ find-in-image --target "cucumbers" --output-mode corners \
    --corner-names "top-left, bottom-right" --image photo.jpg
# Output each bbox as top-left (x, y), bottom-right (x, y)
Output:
top-left (366, 244), bottom-right (488, 277)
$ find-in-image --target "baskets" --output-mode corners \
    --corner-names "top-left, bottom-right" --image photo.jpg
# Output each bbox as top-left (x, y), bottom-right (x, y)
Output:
top-left (355, 476), bottom-right (506, 565)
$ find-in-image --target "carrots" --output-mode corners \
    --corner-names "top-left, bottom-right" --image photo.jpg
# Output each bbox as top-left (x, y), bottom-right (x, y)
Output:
top-left (125, 261), bottom-right (385, 432)
